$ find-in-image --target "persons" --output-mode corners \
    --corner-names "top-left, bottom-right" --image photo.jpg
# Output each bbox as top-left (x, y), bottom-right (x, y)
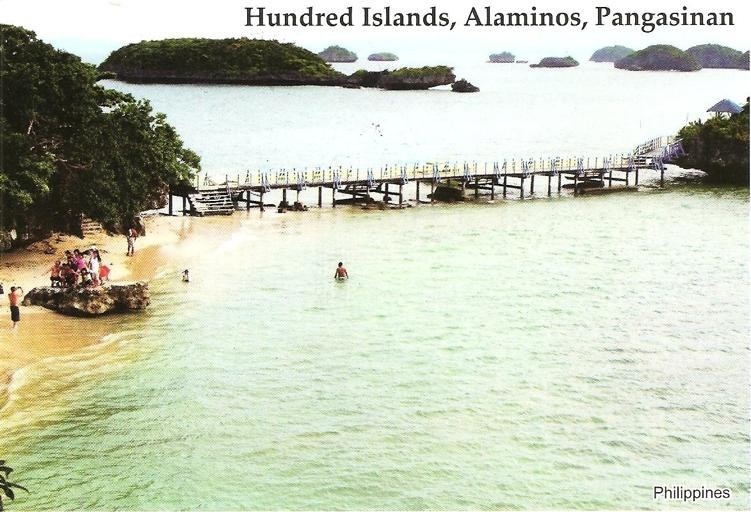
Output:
top-left (183, 270), bottom-right (190, 282)
top-left (8, 285), bottom-right (25, 330)
top-left (335, 262), bottom-right (349, 279)
top-left (42, 247), bottom-right (110, 289)
top-left (126, 225), bottom-right (138, 257)
